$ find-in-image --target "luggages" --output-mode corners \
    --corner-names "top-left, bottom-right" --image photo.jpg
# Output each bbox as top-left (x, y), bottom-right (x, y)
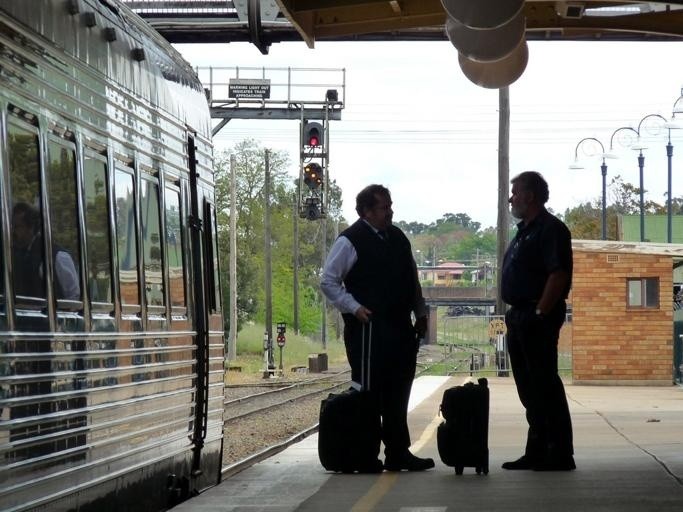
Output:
top-left (437, 379), bottom-right (489, 476)
top-left (317, 313), bottom-right (383, 474)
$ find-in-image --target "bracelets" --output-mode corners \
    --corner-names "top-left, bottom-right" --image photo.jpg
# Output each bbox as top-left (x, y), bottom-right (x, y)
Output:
top-left (536, 307), bottom-right (543, 316)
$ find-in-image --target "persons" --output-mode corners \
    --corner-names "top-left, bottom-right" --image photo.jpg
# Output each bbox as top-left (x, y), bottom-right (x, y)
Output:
top-left (149, 246), bottom-right (173, 272)
top-left (320, 185), bottom-right (434, 474)
top-left (4, 201), bottom-right (79, 458)
top-left (88, 266), bottom-right (103, 302)
top-left (501, 171), bottom-right (576, 471)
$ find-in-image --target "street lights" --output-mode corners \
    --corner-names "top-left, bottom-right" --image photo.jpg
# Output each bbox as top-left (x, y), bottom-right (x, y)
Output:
top-left (667, 88), bottom-right (683, 132)
top-left (604, 125), bottom-right (646, 242)
top-left (484, 261), bottom-right (493, 289)
top-left (632, 114), bottom-right (673, 242)
top-left (569, 137), bottom-right (608, 240)
top-left (424, 258), bottom-right (444, 287)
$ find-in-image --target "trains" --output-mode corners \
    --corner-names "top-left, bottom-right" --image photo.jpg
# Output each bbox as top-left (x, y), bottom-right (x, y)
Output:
top-left (0, 0), bottom-right (225, 512)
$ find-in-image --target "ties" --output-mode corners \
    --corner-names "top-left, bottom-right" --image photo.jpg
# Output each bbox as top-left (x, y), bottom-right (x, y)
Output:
top-left (379, 229), bottom-right (386, 239)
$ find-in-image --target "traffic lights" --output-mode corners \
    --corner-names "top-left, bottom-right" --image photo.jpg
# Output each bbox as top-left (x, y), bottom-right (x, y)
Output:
top-left (309, 127), bottom-right (319, 148)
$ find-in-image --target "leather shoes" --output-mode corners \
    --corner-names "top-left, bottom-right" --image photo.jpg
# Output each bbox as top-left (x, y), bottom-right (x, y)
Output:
top-left (382, 448), bottom-right (435, 472)
top-left (502, 454), bottom-right (575, 471)
